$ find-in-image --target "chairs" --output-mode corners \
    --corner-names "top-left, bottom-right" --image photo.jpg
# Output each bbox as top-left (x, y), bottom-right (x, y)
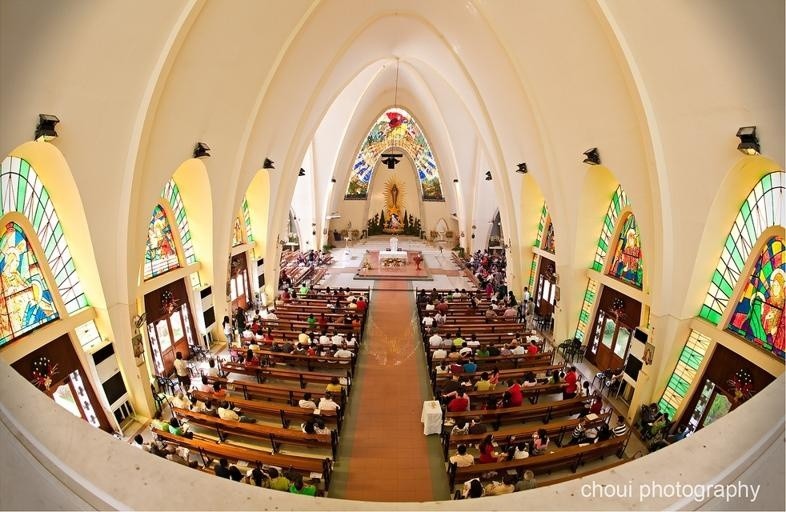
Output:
top-left (639, 404), bottom-right (676, 442)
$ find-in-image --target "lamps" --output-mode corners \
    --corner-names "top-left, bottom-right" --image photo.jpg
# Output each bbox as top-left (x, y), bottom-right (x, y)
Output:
top-left (485, 171), bottom-right (492, 181)
top-left (381, 56), bottom-right (403, 169)
top-left (582, 147), bottom-right (601, 166)
top-left (515, 163), bottom-right (528, 174)
top-left (733, 126), bottom-right (762, 156)
top-left (193, 142), bottom-right (211, 161)
top-left (34, 113), bottom-right (60, 144)
top-left (263, 158), bottom-right (276, 170)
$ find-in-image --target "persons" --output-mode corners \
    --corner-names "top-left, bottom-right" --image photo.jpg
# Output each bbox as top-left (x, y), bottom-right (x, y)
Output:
top-left (391, 184), bottom-right (398, 208)
top-left (130, 249), bottom-right (688, 503)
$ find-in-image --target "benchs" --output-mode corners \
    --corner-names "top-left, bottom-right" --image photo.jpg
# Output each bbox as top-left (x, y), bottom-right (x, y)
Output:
top-left (142, 287), bottom-right (369, 496)
top-left (414, 283), bottom-right (645, 499)
top-left (280, 249), bottom-right (334, 287)
top-left (449, 252), bottom-right (506, 287)
